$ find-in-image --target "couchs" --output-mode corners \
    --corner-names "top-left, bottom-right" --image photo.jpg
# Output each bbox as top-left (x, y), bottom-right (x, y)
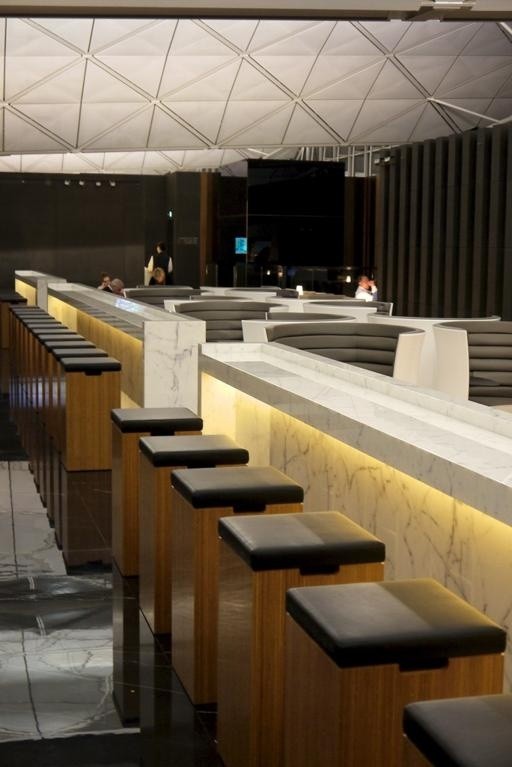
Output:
top-left (303, 299), bottom-right (394, 322)
top-left (365, 311), bottom-right (500, 385)
top-left (266, 323), bottom-right (425, 386)
top-left (172, 302), bottom-right (289, 343)
top-left (433, 322), bottom-right (511, 413)
top-left (242, 312), bottom-right (355, 345)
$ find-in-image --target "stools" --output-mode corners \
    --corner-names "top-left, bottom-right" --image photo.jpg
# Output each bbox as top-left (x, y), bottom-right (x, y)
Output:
top-left (167, 463), bottom-right (304, 707)
top-left (110, 407), bottom-right (202, 578)
top-left (284, 585), bottom-right (506, 764)
top-left (137, 434), bottom-right (250, 635)
top-left (217, 516), bottom-right (386, 766)
top-left (3, 291), bottom-right (123, 470)
top-left (405, 689), bottom-right (510, 767)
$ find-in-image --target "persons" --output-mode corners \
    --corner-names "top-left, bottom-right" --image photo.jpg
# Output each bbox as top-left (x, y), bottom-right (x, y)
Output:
top-left (148, 241), bottom-right (173, 277)
top-left (355, 275), bottom-right (378, 302)
top-left (111, 279), bottom-right (124, 297)
top-left (97, 273), bottom-right (113, 292)
top-left (149, 268), bottom-right (172, 287)
